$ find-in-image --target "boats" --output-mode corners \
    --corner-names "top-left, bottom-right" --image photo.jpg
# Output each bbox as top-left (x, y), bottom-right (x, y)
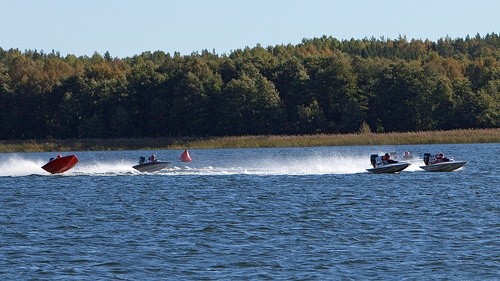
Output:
top-left (365, 162), bottom-right (412, 173)
top-left (180, 149), bottom-right (192, 162)
top-left (132, 160), bottom-right (171, 173)
top-left (41, 154), bottom-right (79, 174)
top-left (419, 161), bottom-right (467, 172)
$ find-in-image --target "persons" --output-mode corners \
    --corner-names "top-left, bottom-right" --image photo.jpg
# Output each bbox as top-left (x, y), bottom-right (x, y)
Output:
top-left (149, 150), bottom-right (445, 166)
